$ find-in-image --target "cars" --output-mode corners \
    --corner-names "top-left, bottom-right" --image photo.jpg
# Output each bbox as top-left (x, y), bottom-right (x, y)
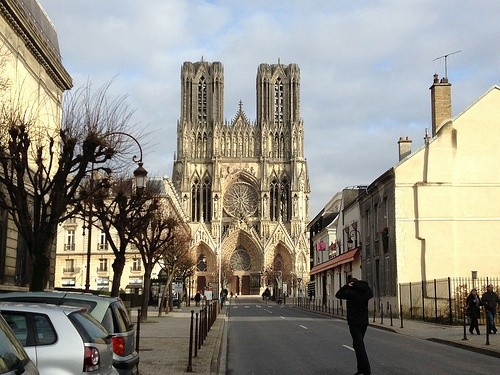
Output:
top-left (0, 316), bottom-right (40, 375)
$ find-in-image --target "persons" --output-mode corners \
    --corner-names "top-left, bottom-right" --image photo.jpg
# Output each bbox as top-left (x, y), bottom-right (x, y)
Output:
top-left (464, 288), bottom-right (487, 336)
top-left (335, 277), bottom-right (374, 375)
top-left (480, 284), bottom-right (500, 334)
top-left (192, 286), bottom-right (238, 308)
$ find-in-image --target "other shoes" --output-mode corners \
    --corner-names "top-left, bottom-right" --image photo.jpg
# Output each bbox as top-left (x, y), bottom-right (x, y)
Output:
top-left (469, 330), bottom-right (473, 334)
top-left (489, 331), bottom-right (492, 334)
top-left (493, 329), bottom-right (497, 334)
top-left (477, 332), bottom-right (480, 335)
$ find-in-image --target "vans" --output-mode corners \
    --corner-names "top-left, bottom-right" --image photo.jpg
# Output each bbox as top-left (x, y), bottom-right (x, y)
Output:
top-left (0, 290), bottom-right (139, 375)
top-left (0, 300), bottom-right (120, 375)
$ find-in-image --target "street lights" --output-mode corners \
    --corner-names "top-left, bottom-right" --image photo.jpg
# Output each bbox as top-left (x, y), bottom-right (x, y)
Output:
top-left (86, 129), bottom-right (149, 287)
top-left (347, 229), bottom-right (362, 248)
